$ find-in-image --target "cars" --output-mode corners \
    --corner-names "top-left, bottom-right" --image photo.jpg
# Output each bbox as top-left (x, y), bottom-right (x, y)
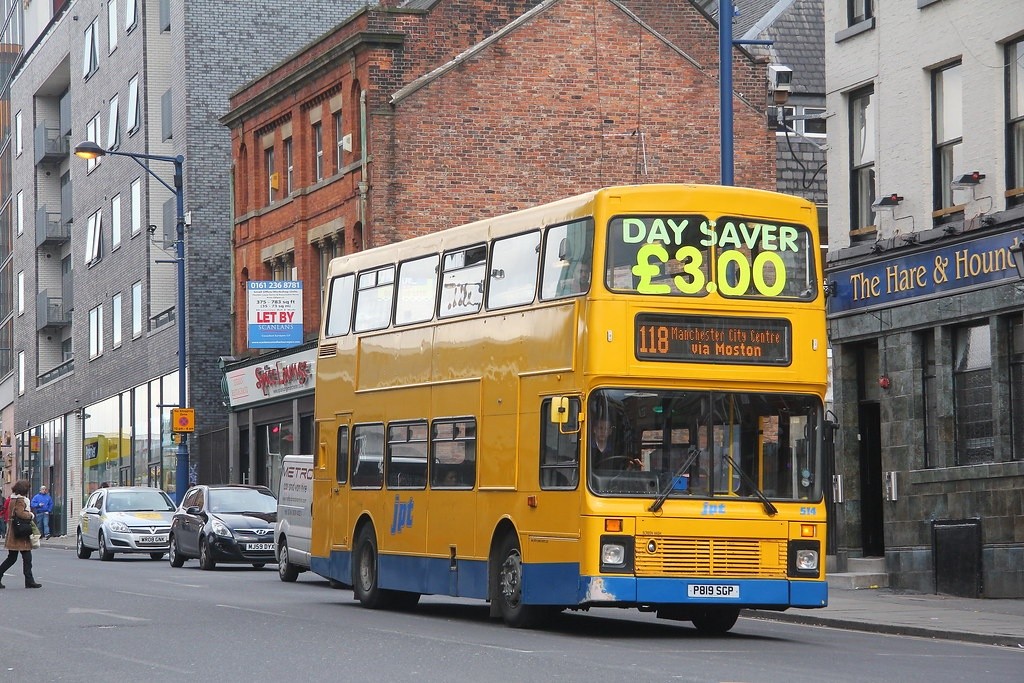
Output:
top-left (76, 486), bottom-right (178, 560)
top-left (169, 484), bottom-right (277, 571)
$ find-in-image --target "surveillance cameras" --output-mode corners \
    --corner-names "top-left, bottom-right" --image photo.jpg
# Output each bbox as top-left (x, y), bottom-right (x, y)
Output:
top-left (767, 65), bottom-right (793, 85)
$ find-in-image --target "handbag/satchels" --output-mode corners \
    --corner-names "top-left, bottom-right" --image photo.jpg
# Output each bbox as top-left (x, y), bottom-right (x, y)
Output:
top-left (12, 496), bottom-right (32, 537)
top-left (30, 534), bottom-right (41, 549)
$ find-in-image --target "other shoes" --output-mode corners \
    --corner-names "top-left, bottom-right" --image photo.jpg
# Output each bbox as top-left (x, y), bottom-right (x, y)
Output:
top-left (45, 534), bottom-right (50, 540)
top-left (25, 582), bottom-right (42, 588)
top-left (0, 582), bottom-right (5, 588)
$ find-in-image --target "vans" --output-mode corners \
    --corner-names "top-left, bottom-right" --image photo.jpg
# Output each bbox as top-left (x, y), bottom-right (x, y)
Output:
top-left (274, 453), bottom-right (313, 581)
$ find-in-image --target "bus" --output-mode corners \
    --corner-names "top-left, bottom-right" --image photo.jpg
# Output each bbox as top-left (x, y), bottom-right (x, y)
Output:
top-left (311, 185), bottom-right (828, 634)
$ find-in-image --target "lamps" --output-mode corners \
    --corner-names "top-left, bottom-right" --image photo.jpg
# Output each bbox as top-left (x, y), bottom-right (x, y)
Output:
top-left (950, 173), bottom-right (985, 190)
top-left (871, 194), bottom-right (903, 210)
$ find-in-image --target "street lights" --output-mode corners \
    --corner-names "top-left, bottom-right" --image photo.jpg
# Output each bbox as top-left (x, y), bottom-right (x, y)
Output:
top-left (74, 142), bottom-right (190, 508)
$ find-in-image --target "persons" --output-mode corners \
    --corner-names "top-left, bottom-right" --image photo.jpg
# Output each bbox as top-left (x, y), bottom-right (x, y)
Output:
top-left (0, 489), bottom-right (10, 539)
top-left (437, 467), bottom-right (459, 485)
top-left (100, 482), bottom-right (109, 488)
top-left (736, 266), bottom-right (758, 295)
top-left (0, 479), bottom-right (42, 588)
top-left (591, 413), bottom-right (644, 470)
top-left (562, 257), bottom-right (590, 295)
top-left (31, 485), bottom-right (54, 540)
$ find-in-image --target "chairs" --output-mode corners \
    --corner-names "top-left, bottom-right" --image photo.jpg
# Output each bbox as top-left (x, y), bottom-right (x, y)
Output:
top-left (351, 472), bottom-right (426, 487)
